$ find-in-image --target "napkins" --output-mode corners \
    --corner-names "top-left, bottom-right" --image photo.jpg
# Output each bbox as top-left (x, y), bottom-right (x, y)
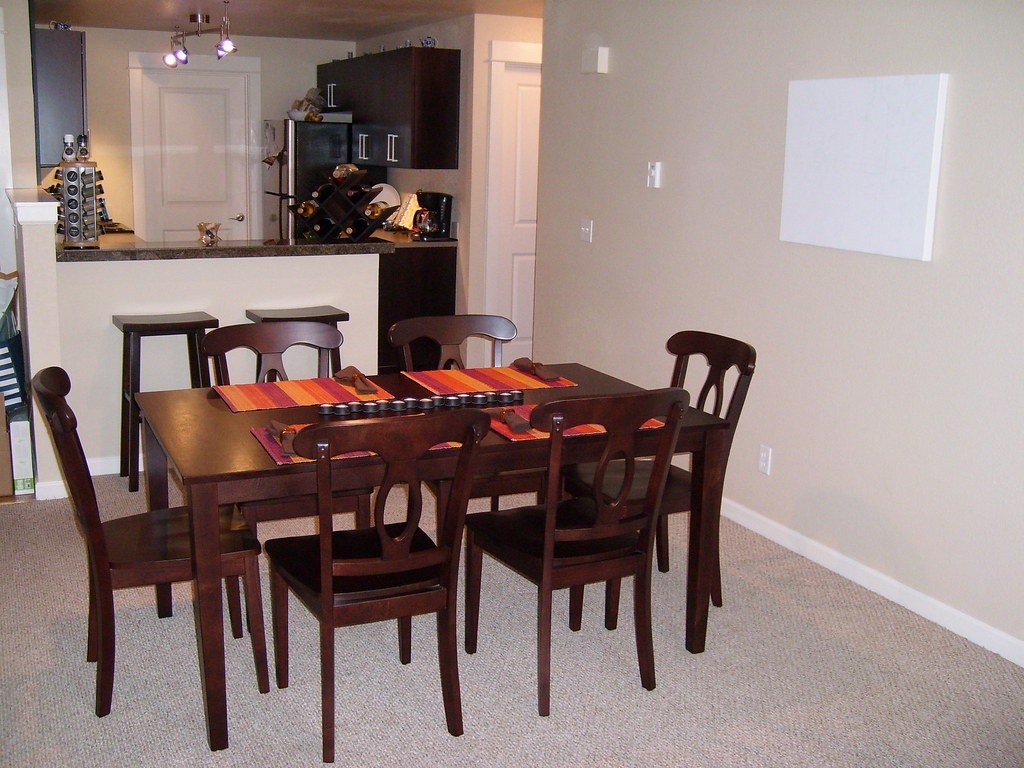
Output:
top-left (482, 407), bottom-right (530, 433)
top-left (266, 420), bottom-right (297, 455)
top-left (513, 357), bottom-right (559, 381)
top-left (335, 366), bottom-right (378, 393)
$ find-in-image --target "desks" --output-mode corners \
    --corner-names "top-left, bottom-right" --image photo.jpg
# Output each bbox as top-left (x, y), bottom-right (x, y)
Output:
top-left (126, 362), bottom-right (731, 752)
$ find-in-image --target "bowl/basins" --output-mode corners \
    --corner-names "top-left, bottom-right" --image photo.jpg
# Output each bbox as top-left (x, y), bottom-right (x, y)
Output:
top-left (288, 111), bottom-right (308, 122)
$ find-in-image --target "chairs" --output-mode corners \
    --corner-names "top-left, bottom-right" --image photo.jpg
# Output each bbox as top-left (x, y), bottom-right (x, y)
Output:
top-left (31, 314), bottom-right (758, 763)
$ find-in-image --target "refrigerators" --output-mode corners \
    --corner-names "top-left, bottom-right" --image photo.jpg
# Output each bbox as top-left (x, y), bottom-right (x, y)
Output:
top-left (282, 119), bottom-right (350, 240)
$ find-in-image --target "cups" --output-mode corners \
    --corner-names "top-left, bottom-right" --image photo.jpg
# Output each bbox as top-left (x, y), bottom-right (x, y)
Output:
top-left (348, 52), bottom-right (353, 59)
top-left (406, 40), bottom-right (410, 47)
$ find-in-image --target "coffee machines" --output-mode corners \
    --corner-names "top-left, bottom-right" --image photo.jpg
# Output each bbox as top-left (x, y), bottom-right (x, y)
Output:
top-left (411, 192), bottom-right (452, 241)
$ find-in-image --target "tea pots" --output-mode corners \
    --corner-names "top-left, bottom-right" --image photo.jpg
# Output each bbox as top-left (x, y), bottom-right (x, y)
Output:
top-left (419, 37), bottom-right (439, 48)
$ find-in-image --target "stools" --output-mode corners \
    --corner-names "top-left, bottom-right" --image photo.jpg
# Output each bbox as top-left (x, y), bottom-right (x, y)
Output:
top-left (246, 305), bottom-right (349, 383)
top-left (112, 311), bottom-right (219, 492)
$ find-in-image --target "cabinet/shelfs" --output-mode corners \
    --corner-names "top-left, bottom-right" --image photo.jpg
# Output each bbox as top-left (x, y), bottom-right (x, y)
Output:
top-left (30, 28), bottom-right (88, 168)
top-left (317, 46), bottom-right (461, 170)
top-left (287, 170), bottom-right (401, 243)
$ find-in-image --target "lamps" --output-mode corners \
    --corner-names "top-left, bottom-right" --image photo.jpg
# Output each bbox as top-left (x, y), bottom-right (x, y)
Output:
top-left (223, 0), bottom-right (237, 53)
top-left (159, 13), bottom-right (234, 68)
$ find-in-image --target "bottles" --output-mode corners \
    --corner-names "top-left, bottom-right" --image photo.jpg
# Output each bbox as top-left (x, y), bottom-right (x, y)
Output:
top-left (365, 202), bottom-right (388, 219)
top-left (333, 164), bottom-right (359, 179)
top-left (311, 192), bottom-right (328, 199)
top-left (348, 186), bottom-right (367, 197)
top-left (298, 202), bottom-right (317, 217)
top-left (314, 219), bottom-right (335, 234)
top-left (380, 41), bottom-right (385, 52)
top-left (346, 219), bottom-right (366, 235)
